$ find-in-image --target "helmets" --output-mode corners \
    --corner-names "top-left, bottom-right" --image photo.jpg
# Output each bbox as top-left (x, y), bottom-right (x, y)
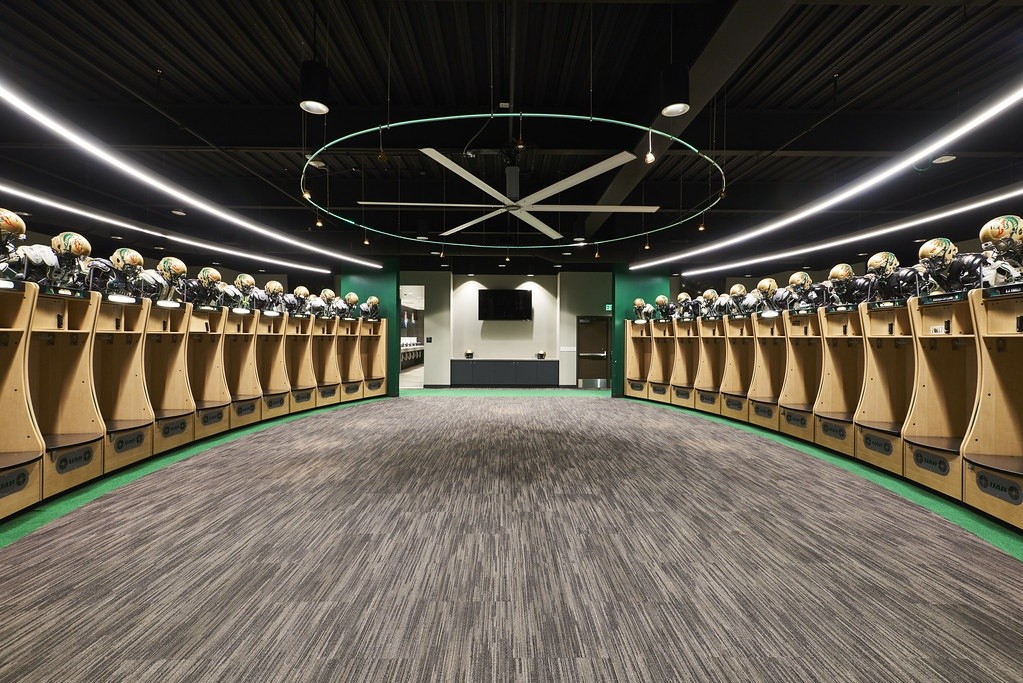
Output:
top-left (828, 264), bottom-right (854, 283)
top-left (918, 238), bottom-right (959, 270)
top-left (367, 296), bottom-right (379, 310)
top-left (294, 286), bottom-right (310, 303)
top-left (678, 293), bottom-right (691, 307)
top-left (867, 251), bottom-right (899, 280)
top-left (0, 207), bottom-right (26, 247)
top-left (979, 215), bottom-right (1023, 261)
top-left (756, 277), bottom-right (779, 299)
top-left (157, 257), bottom-right (187, 286)
top-left (634, 299), bottom-right (646, 310)
top-left (51, 232), bottom-right (92, 264)
top-left (234, 274), bottom-right (255, 295)
top-left (655, 294), bottom-right (669, 308)
top-left (198, 267), bottom-right (221, 290)
top-left (703, 289), bottom-right (719, 306)
top-left (789, 271), bottom-right (812, 295)
top-left (110, 248), bottom-right (144, 271)
top-left (345, 293), bottom-right (359, 306)
top-left (265, 280), bottom-right (284, 302)
top-left (730, 283), bottom-right (747, 298)
top-left (320, 289), bottom-right (336, 305)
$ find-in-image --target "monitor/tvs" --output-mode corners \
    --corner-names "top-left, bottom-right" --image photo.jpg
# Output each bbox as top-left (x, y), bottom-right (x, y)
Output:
top-left (478, 289), bottom-right (532, 320)
